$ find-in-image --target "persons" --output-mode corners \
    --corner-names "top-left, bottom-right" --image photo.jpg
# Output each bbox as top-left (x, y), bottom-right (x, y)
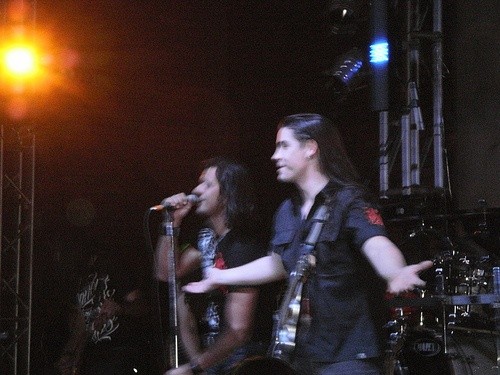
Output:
top-left (454, 220), bottom-right (499, 270)
top-left (57, 230), bottom-right (154, 374)
top-left (183, 112), bottom-right (435, 375)
top-left (152, 156), bottom-right (278, 375)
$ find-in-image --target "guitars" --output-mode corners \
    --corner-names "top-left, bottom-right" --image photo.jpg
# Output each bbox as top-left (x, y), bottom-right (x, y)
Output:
top-left (270, 253), bottom-right (316, 360)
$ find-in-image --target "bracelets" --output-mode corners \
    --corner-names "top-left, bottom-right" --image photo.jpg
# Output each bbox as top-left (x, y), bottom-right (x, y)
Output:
top-left (161, 227), bottom-right (181, 236)
top-left (190, 358), bottom-right (204, 375)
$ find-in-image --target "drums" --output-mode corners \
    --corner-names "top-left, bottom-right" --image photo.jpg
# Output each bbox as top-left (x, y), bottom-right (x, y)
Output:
top-left (404, 328), bottom-right (497, 375)
top-left (452, 273), bottom-right (492, 317)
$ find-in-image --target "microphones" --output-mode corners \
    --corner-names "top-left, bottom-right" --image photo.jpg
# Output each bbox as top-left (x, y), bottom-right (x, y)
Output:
top-left (150, 194), bottom-right (199, 211)
